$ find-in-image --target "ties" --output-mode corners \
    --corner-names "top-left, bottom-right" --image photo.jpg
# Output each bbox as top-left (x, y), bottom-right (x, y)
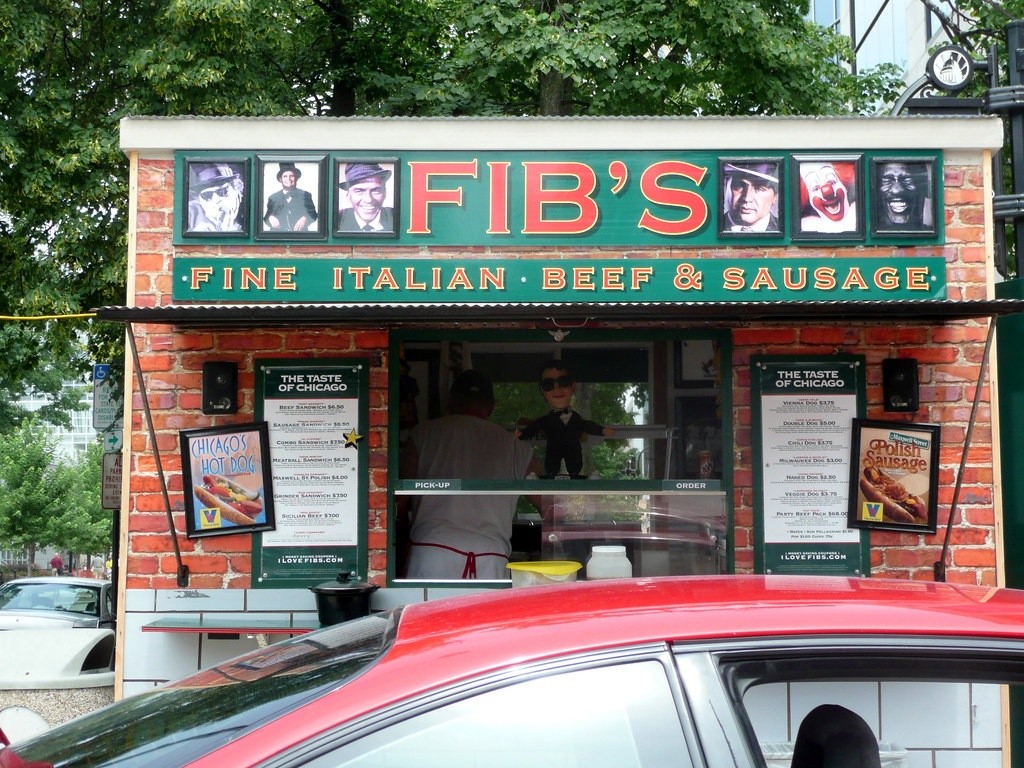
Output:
top-left (362, 225), bottom-right (374, 231)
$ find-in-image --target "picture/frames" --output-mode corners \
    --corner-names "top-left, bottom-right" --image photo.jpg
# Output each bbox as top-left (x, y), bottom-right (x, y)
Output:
top-left (332, 157), bottom-right (401, 239)
top-left (847, 418), bottom-right (941, 535)
top-left (253, 154), bottom-right (329, 243)
top-left (790, 153), bottom-right (866, 242)
top-left (869, 156), bottom-right (939, 239)
top-left (179, 420), bottom-right (277, 539)
top-left (717, 156), bottom-right (786, 239)
top-left (183, 156), bottom-right (251, 238)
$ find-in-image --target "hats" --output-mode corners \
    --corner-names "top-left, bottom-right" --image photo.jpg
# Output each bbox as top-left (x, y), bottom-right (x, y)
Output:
top-left (339, 162), bottom-right (392, 191)
top-left (723, 163), bottom-right (779, 183)
top-left (277, 163), bottom-right (301, 182)
top-left (191, 163), bottom-right (239, 191)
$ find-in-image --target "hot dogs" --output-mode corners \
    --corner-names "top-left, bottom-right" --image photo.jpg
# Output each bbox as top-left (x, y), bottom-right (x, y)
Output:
top-left (859, 465), bottom-right (928, 523)
top-left (194, 474), bottom-right (264, 525)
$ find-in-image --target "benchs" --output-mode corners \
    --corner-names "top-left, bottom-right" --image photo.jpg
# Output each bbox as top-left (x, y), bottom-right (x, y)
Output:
top-left (141, 619), bottom-right (320, 670)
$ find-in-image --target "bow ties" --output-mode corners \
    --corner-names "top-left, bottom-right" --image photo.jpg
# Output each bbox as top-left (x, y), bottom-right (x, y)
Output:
top-left (284, 192), bottom-right (294, 199)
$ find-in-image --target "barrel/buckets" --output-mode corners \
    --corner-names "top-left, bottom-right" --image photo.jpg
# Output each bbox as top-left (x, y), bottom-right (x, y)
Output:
top-left (504, 561), bottom-right (582, 591)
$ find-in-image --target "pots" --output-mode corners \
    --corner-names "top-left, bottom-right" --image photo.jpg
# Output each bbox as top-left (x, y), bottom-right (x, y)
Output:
top-left (307, 572), bottom-right (381, 626)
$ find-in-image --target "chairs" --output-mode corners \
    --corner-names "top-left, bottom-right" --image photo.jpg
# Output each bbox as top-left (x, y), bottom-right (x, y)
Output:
top-left (790, 704), bottom-right (882, 768)
top-left (32, 597), bottom-right (53, 607)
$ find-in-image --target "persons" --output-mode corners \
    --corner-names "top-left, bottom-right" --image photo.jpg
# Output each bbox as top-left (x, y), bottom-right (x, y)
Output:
top-left (514, 358), bottom-right (616, 480)
top-left (188, 163), bottom-right (244, 232)
top-left (90, 552), bottom-right (112, 580)
top-left (395, 369), bottom-right (566, 579)
top-left (338, 163), bottom-right (394, 231)
top-left (722, 161), bottom-right (938, 233)
top-left (263, 163), bottom-right (318, 231)
top-left (49, 553), bottom-right (65, 576)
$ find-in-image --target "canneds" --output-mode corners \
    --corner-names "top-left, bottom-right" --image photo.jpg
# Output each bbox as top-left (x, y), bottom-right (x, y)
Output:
top-left (695, 450), bottom-right (714, 479)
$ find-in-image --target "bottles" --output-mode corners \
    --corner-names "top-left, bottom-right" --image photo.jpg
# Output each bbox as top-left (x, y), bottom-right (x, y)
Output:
top-left (586, 545), bottom-right (632, 580)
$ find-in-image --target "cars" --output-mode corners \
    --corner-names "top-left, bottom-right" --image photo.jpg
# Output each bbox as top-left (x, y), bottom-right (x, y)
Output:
top-left (0, 573), bottom-right (1024, 768)
top-left (0, 576), bottom-right (116, 692)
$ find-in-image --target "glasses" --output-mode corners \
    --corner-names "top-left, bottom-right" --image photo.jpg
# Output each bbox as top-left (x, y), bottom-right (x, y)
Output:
top-left (198, 182), bottom-right (230, 201)
